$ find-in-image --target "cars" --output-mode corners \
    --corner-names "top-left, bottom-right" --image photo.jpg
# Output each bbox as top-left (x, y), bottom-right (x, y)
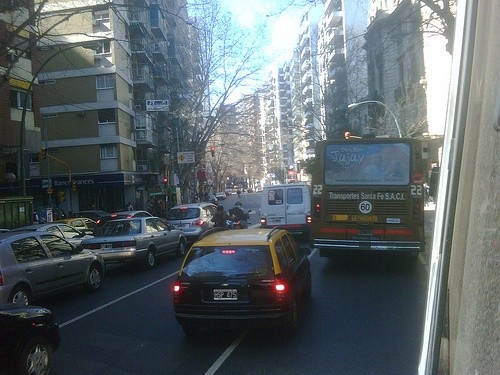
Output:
top-left (0, 231), bottom-right (105, 305)
top-left (12, 209), bottom-right (153, 238)
top-left (167, 202), bottom-right (219, 237)
top-left (82, 216), bottom-right (187, 271)
top-left (216, 192), bottom-right (227, 199)
top-left (0, 304), bottom-right (62, 375)
top-left (172, 226), bottom-right (312, 336)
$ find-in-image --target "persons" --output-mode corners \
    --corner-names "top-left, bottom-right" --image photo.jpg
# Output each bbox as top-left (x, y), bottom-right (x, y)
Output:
top-left (211, 205), bottom-right (229, 230)
top-left (237, 188), bottom-right (245, 198)
top-left (230, 202), bottom-right (249, 229)
top-left (127, 203), bottom-right (134, 210)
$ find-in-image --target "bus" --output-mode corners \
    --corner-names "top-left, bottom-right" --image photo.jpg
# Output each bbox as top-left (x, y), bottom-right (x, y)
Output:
top-left (311, 133), bottom-right (425, 260)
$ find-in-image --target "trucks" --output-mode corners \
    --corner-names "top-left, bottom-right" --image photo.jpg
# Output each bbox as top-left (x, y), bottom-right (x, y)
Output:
top-left (259, 184), bottom-right (311, 231)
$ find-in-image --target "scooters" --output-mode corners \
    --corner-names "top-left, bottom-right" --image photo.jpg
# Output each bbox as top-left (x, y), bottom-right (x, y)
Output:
top-left (229, 210), bottom-right (252, 229)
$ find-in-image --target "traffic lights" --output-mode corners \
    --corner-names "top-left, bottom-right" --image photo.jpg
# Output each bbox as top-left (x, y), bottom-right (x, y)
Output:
top-left (211, 146), bottom-right (215, 157)
top-left (41, 146), bottom-right (47, 159)
top-left (59, 191), bottom-right (66, 204)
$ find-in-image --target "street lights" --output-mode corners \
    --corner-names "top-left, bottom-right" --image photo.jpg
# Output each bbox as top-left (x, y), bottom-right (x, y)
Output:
top-left (348, 99), bottom-right (403, 139)
top-left (20, 40), bottom-right (101, 197)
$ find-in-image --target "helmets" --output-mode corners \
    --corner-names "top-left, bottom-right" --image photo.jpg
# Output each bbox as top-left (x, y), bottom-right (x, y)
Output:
top-left (235, 202), bottom-right (242, 206)
top-left (217, 205), bottom-right (223, 209)
